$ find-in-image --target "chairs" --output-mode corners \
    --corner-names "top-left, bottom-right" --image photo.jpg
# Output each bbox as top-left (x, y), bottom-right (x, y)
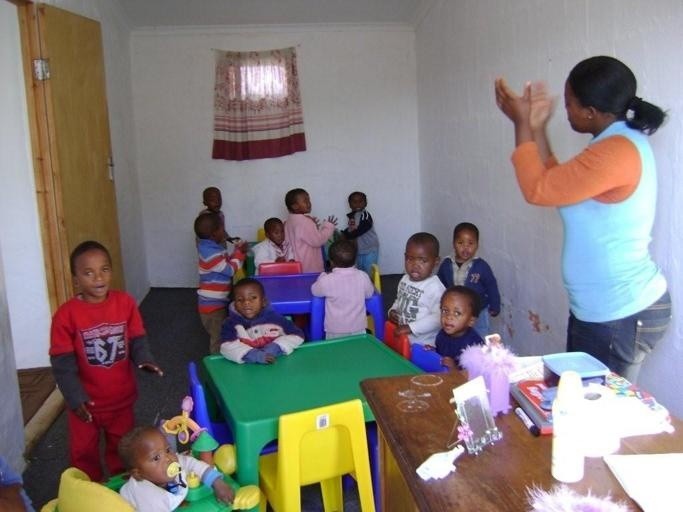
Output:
top-left (383, 318), bottom-right (416, 359)
top-left (363, 264), bottom-right (381, 337)
top-left (187, 361), bottom-right (279, 456)
top-left (256, 228), bottom-right (268, 241)
top-left (257, 397), bottom-right (376, 511)
top-left (258, 262), bottom-right (307, 328)
top-left (343, 341), bottom-right (444, 511)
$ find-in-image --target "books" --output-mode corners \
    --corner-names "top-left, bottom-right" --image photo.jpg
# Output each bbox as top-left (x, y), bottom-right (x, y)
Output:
top-left (509, 349), bottom-right (671, 436)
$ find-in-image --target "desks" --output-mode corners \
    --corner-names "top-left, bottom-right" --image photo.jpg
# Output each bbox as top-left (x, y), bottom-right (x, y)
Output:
top-left (196, 332), bottom-right (429, 511)
top-left (245, 240), bottom-right (262, 274)
top-left (245, 271), bottom-right (386, 343)
top-left (359, 351), bottom-right (682, 511)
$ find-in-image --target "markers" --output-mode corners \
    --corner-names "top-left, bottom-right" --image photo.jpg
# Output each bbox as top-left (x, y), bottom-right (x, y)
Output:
top-left (514, 406), bottom-right (539, 437)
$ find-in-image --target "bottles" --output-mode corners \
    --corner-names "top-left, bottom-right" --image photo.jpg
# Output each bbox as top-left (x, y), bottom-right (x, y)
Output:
top-left (550, 373), bottom-right (586, 480)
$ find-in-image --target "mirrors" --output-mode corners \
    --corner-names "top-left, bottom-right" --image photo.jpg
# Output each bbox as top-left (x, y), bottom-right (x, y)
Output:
top-left (444, 373), bottom-right (503, 458)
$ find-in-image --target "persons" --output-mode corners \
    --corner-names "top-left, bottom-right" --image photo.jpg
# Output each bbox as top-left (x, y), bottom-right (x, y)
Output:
top-left (194, 187), bottom-right (380, 365)
top-left (118, 425), bottom-right (236, 512)
top-left (50, 241), bottom-right (165, 484)
top-left (387, 222), bottom-right (501, 372)
top-left (496, 56), bottom-right (674, 384)
top-left (0, 454), bottom-right (40, 511)
